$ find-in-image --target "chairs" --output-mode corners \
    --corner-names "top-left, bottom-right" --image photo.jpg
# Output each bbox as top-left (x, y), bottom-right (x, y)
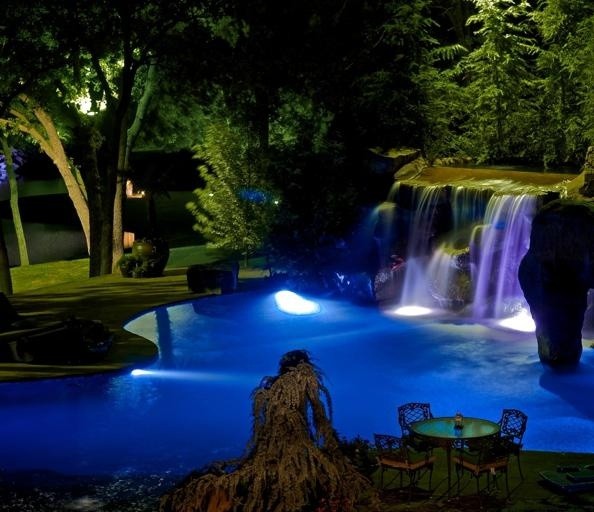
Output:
top-left (452, 434), bottom-right (516, 512)
top-left (486, 408), bottom-right (527, 480)
top-left (372, 433), bottom-right (437, 490)
top-left (397, 402), bottom-right (436, 479)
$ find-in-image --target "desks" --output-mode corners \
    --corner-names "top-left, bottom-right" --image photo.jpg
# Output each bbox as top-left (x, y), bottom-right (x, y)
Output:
top-left (409, 415), bottom-right (502, 501)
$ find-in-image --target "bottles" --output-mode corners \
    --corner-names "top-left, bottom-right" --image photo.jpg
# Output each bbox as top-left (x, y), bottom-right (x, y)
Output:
top-left (454, 411), bottom-right (463, 430)
